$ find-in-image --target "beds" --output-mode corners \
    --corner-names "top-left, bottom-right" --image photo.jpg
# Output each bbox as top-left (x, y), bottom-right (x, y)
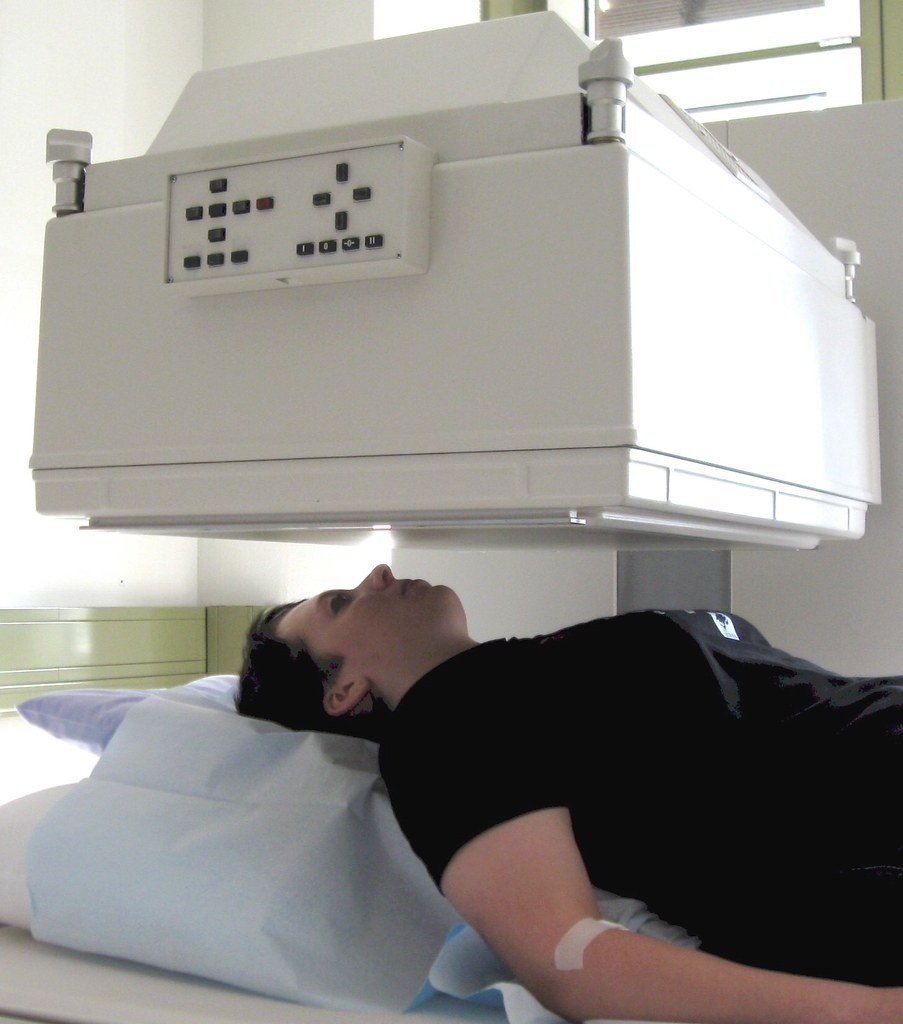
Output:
top-left (1, 708), bottom-right (709, 1024)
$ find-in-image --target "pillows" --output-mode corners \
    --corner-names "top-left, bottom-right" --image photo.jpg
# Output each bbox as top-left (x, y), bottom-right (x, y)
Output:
top-left (17, 674), bottom-right (244, 757)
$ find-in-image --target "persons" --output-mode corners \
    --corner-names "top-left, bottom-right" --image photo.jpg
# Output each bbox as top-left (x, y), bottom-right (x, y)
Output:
top-left (233, 564), bottom-right (903, 1024)
